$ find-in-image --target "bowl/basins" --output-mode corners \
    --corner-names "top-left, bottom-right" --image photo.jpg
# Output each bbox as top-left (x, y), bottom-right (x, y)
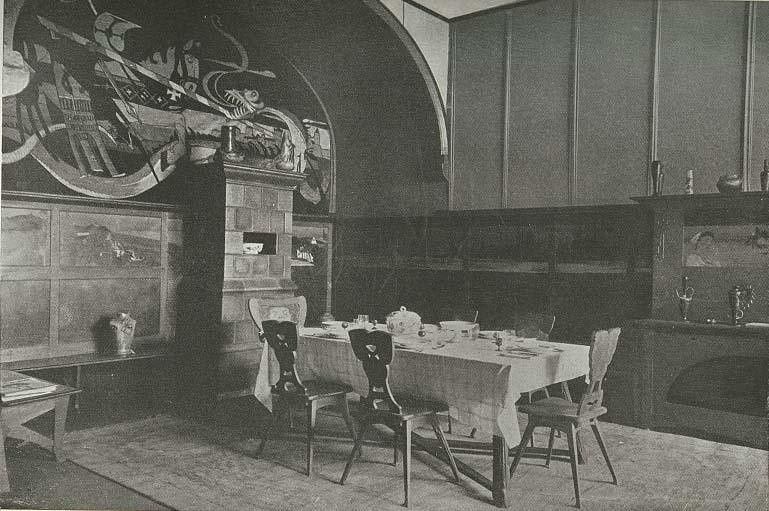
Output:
top-left (243, 243), bottom-right (264, 255)
top-left (321, 321), bottom-right (347, 336)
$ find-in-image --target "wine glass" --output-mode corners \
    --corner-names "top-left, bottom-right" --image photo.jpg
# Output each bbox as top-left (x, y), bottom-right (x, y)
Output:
top-left (341, 323), bottom-right (349, 337)
top-left (418, 326), bottom-right (426, 344)
top-left (372, 320), bottom-right (378, 329)
top-left (495, 338), bottom-right (503, 353)
top-left (438, 321), bottom-right (477, 344)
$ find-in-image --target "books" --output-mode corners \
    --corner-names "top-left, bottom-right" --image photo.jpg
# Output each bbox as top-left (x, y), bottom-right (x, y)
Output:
top-left (0, 376), bottom-right (57, 402)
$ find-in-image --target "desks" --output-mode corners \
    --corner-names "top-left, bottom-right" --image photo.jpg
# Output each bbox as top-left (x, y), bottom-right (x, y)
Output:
top-left (253, 321), bottom-right (591, 509)
top-left (0, 366), bottom-right (83, 495)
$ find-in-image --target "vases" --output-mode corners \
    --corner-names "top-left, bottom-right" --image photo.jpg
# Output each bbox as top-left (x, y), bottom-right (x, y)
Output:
top-left (651, 159), bottom-right (664, 198)
top-left (109, 310), bottom-right (137, 357)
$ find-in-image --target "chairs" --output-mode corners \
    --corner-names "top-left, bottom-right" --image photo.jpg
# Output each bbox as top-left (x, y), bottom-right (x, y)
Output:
top-left (507, 327), bottom-right (622, 508)
top-left (247, 295), bottom-right (561, 449)
top-left (254, 320), bottom-right (364, 477)
top-left (340, 329), bottom-right (462, 508)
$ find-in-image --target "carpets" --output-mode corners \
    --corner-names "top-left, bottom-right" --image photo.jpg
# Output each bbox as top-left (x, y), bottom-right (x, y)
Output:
top-left (63, 408), bottom-right (769, 511)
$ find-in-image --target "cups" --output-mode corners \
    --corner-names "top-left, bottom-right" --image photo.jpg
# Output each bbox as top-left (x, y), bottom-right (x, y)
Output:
top-left (466, 324), bottom-right (480, 347)
top-left (358, 315), bottom-right (369, 330)
top-left (502, 330), bottom-right (516, 349)
